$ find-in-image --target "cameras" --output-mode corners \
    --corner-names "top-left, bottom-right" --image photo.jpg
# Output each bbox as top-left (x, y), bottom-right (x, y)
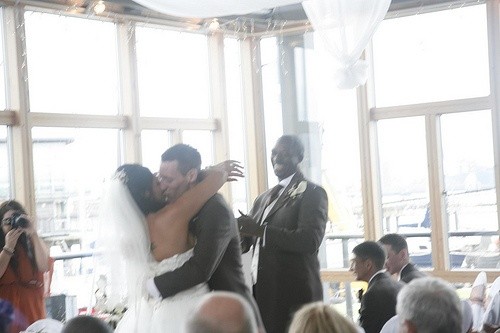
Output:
top-left (8, 213), bottom-right (27, 230)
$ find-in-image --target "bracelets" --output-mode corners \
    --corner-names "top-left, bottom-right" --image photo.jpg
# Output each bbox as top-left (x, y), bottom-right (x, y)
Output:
top-left (3, 245), bottom-right (15, 255)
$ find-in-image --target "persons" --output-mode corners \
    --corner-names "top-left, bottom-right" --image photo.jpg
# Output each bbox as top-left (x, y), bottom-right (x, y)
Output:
top-left (380, 272), bottom-right (500, 333)
top-left (62, 315), bottom-right (113, 333)
top-left (377, 233), bottom-right (427, 284)
top-left (237, 135), bottom-right (328, 333)
top-left (21, 318), bottom-right (65, 333)
top-left (0, 200), bottom-right (54, 333)
top-left (349, 241), bottom-right (403, 333)
top-left (103, 159), bottom-right (245, 333)
top-left (289, 301), bottom-right (360, 333)
top-left (146, 143), bottom-right (267, 333)
top-left (183, 292), bottom-right (259, 333)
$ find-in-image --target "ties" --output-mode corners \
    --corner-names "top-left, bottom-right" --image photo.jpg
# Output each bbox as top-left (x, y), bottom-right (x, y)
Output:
top-left (265, 185), bottom-right (282, 208)
